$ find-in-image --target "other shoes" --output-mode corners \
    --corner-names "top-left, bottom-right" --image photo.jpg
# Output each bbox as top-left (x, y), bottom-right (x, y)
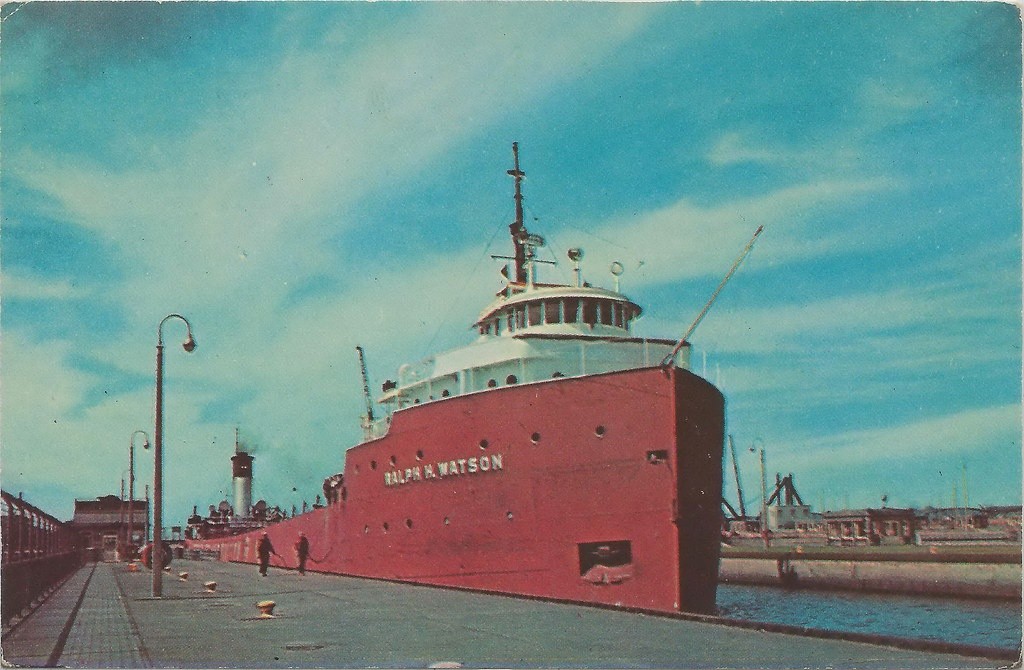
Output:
top-left (298, 571), bottom-right (305, 576)
top-left (262, 573), bottom-right (268, 576)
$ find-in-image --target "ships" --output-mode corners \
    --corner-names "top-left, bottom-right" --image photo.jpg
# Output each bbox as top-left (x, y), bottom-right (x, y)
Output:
top-left (170, 143), bottom-right (764, 618)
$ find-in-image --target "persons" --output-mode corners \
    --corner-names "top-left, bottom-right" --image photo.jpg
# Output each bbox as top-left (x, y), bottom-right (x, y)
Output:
top-left (295, 531), bottom-right (310, 575)
top-left (257, 531), bottom-right (278, 576)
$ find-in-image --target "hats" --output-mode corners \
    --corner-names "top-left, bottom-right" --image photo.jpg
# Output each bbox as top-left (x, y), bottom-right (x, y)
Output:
top-left (261, 531), bottom-right (268, 536)
top-left (298, 531), bottom-right (305, 537)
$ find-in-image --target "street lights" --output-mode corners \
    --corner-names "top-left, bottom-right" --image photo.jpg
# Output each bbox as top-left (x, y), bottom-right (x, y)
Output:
top-left (145, 313), bottom-right (202, 600)
top-left (126, 429), bottom-right (151, 564)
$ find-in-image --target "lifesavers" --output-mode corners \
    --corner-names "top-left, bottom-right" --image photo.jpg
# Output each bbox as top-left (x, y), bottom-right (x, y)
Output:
top-left (762, 530), bottom-right (773, 540)
top-left (611, 261), bottom-right (624, 275)
top-left (142, 542), bottom-right (173, 569)
